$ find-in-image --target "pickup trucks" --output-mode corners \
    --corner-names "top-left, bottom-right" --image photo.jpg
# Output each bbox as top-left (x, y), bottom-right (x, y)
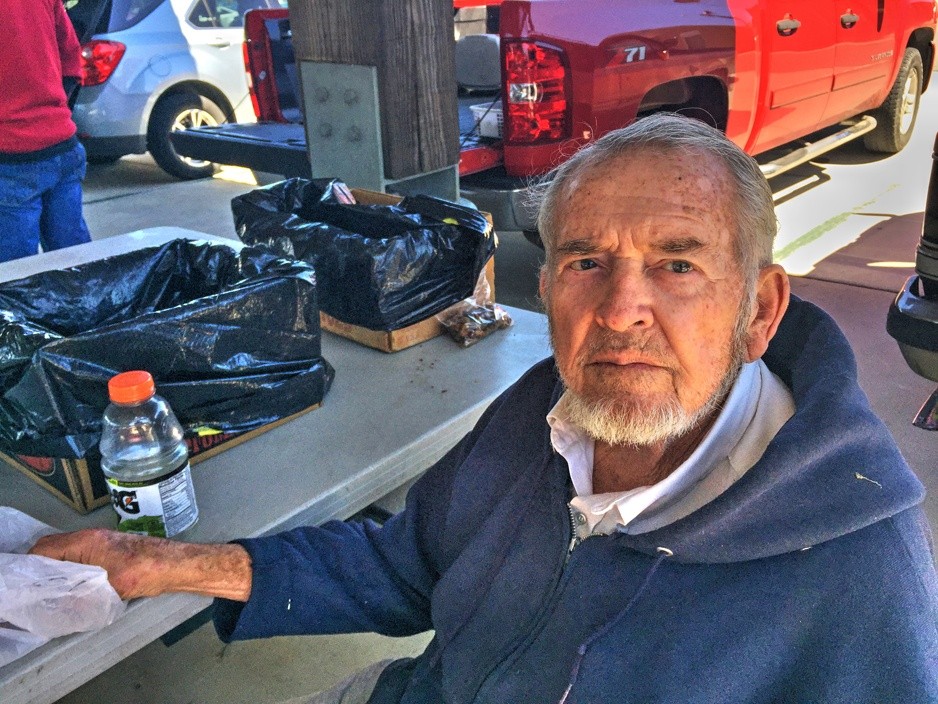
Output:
top-left (165, 0), bottom-right (934, 250)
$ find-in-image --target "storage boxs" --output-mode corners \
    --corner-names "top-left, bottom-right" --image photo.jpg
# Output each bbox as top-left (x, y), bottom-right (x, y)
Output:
top-left (0, 239), bottom-right (335, 514)
top-left (229, 175), bottom-right (498, 353)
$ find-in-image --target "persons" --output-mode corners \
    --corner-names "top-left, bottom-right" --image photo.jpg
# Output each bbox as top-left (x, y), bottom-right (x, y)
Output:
top-left (28, 110), bottom-right (938, 703)
top-left (0, 0), bottom-right (93, 263)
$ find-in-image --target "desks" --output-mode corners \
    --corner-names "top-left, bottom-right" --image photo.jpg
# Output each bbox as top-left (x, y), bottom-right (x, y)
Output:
top-left (0, 226), bottom-right (557, 704)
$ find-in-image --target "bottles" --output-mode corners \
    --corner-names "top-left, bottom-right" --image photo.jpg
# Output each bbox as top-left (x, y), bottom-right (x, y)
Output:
top-left (100, 370), bottom-right (199, 540)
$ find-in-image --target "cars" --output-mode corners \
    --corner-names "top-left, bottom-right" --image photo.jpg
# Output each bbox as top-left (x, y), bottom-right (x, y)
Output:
top-left (64, 0), bottom-right (291, 180)
